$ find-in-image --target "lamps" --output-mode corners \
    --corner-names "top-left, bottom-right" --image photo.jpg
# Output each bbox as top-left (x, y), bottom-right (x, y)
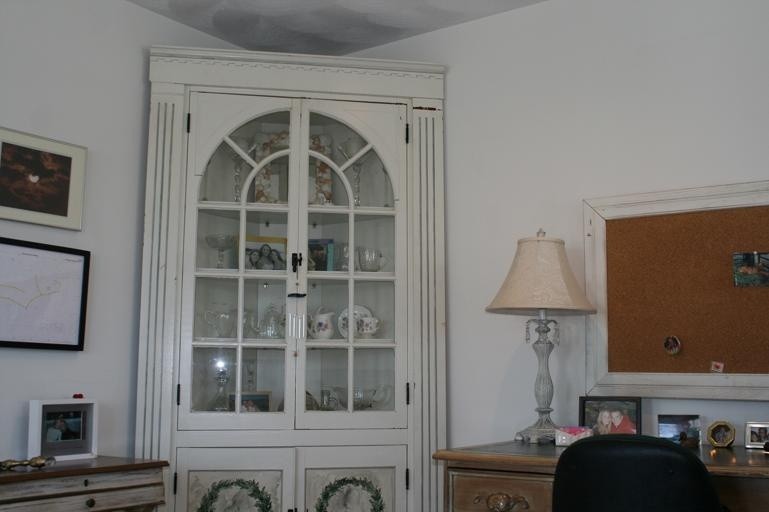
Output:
top-left (485, 228), bottom-right (597, 445)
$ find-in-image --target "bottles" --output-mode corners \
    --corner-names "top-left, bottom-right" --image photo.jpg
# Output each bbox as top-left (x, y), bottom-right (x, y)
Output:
top-left (207, 370), bottom-right (234, 410)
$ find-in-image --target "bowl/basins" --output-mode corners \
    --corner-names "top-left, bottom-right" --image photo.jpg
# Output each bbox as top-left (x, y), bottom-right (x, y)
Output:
top-left (337, 388), bottom-right (376, 410)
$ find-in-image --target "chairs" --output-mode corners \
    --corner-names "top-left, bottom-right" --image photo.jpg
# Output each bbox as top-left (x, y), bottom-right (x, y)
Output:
top-left (552, 434), bottom-right (719, 511)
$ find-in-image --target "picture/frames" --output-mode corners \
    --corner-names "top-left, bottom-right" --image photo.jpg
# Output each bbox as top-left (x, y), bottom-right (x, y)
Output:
top-left (707, 421), bottom-right (735, 448)
top-left (0, 125), bottom-right (88, 232)
top-left (28, 399), bottom-right (98, 462)
top-left (582, 180), bottom-right (769, 401)
top-left (0, 236), bottom-right (91, 351)
top-left (578, 396), bottom-right (642, 436)
top-left (744, 422), bottom-right (769, 449)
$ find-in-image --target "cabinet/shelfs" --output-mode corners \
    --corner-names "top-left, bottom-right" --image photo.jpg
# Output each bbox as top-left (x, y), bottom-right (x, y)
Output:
top-left (133, 43), bottom-right (448, 511)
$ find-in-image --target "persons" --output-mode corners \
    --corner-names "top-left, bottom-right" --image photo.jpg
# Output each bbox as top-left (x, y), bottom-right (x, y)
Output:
top-left (751, 427), bottom-right (768, 442)
top-left (247, 244), bottom-right (286, 269)
top-left (591, 409), bottom-right (636, 436)
top-left (46, 418), bottom-right (76, 440)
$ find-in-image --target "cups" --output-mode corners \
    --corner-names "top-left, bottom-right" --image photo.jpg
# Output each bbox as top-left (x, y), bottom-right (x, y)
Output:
top-left (204, 233), bottom-right (388, 339)
top-left (320, 391), bottom-right (337, 411)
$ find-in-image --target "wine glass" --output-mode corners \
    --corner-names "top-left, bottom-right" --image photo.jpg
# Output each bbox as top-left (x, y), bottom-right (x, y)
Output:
top-left (220, 139), bottom-right (252, 201)
top-left (338, 141), bottom-right (371, 206)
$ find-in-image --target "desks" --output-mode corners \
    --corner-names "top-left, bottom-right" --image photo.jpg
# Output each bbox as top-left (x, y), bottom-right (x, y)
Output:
top-left (0, 456), bottom-right (170, 512)
top-left (432, 440), bottom-right (769, 512)
